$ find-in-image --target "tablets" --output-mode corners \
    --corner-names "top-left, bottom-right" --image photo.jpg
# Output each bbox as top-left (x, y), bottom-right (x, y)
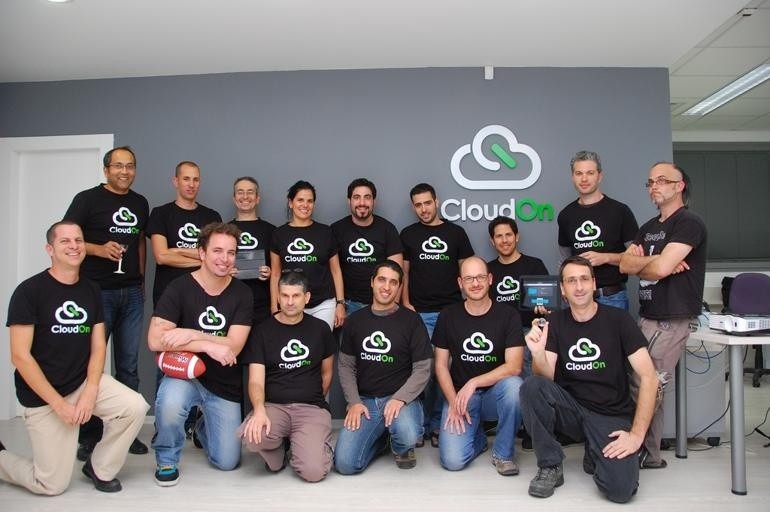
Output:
top-left (233, 248), bottom-right (266, 280)
top-left (518, 275), bottom-right (561, 313)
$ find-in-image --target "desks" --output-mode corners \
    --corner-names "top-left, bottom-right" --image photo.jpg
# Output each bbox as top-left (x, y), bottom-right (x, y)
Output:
top-left (675, 328), bottom-right (770, 495)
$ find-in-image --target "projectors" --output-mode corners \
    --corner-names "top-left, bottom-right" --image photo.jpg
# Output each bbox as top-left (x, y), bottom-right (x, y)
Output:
top-left (709, 312), bottom-right (770, 335)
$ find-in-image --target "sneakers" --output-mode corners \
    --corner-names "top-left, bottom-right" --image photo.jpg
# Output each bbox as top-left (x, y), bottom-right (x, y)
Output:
top-left (265, 437), bottom-right (290, 474)
top-left (186, 405), bottom-right (204, 449)
top-left (154, 463), bottom-right (180, 487)
top-left (582, 440), bottom-right (597, 474)
top-left (639, 459), bottom-right (668, 469)
top-left (130, 437), bottom-right (149, 454)
top-left (394, 448), bottom-right (416, 468)
top-left (491, 453), bottom-right (518, 475)
top-left (76, 443), bottom-right (94, 460)
top-left (528, 461), bottom-right (563, 499)
top-left (82, 461), bottom-right (122, 492)
top-left (520, 436), bottom-right (533, 451)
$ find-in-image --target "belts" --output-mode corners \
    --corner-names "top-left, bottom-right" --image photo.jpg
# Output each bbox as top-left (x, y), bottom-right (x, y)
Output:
top-left (594, 281), bottom-right (626, 300)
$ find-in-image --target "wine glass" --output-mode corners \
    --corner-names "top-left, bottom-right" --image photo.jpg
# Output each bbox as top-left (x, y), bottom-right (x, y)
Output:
top-left (113, 243), bottom-right (129, 274)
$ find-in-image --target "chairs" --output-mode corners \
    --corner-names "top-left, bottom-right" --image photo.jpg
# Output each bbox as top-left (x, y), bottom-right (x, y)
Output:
top-left (724, 273), bottom-right (770, 388)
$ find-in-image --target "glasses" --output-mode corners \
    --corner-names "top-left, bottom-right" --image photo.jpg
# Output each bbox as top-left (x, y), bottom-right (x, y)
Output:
top-left (460, 275), bottom-right (487, 284)
top-left (644, 178), bottom-right (680, 187)
top-left (108, 163), bottom-right (135, 169)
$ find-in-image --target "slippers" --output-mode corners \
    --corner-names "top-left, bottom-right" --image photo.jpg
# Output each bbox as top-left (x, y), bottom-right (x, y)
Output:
top-left (430, 429), bottom-right (439, 447)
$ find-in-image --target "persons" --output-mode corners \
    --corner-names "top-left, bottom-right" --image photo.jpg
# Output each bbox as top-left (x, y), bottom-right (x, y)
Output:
top-left (235, 271), bottom-right (337, 483)
top-left (62, 145), bottom-right (150, 461)
top-left (147, 220), bottom-right (254, 488)
top-left (519, 256), bottom-right (660, 504)
top-left (430, 256), bottom-right (527, 476)
top-left (618, 161), bottom-right (708, 469)
top-left (0, 221), bottom-right (152, 496)
top-left (556, 151), bottom-right (640, 309)
top-left (150, 162), bottom-right (476, 444)
top-left (486, 216), bottom-right (550, 450)
top-left (333, 260), bottom-right (435, 475)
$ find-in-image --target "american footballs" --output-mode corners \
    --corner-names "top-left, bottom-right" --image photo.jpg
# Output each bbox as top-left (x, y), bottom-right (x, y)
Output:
top-left (153, 351), bottom-right (207, 380)
